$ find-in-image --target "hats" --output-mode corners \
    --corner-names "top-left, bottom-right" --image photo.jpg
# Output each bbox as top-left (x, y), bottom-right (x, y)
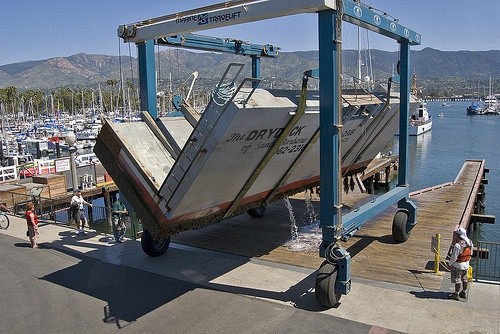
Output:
top-left (455, 228), bottom-right (467, 235)
top-left (74, 189), bottom-right (81, 192)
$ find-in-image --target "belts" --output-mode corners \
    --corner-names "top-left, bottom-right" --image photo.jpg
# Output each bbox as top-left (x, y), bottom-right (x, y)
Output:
top-left (78, 209), bottom-right (84, 211)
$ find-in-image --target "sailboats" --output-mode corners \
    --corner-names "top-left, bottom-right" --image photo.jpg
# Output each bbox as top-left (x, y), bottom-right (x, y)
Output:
top-left (0, 82), bottom-right (140, 182)
top-left (466, 74), bottom-right (500, 116)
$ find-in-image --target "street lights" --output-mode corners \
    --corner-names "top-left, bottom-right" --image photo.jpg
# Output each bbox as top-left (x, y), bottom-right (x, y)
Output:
top-left (64, 131), bottom-right (78, 192)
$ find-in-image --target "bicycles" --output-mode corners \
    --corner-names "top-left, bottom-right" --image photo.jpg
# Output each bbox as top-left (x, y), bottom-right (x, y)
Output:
top-left (0, 209), bottom-right (10, 230)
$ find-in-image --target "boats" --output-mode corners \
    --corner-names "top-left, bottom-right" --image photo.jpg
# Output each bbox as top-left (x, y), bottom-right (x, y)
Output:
top-left (408, 67), bottom-right (433, 135)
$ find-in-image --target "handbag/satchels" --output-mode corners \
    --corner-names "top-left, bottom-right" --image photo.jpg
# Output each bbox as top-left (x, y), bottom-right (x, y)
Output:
top-left (27, 225), bottom-right (30, 236)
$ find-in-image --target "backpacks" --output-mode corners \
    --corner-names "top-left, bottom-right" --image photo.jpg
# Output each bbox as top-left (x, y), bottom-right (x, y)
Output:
top-left (457, 243), bottom-right (471, 263)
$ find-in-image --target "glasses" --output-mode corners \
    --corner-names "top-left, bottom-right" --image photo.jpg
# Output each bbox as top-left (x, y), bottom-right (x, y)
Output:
top-left (32, 207), bottom-right (35, 209)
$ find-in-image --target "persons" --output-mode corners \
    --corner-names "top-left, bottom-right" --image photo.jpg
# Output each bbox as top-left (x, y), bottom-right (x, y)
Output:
top-left (70, 189), bottom-right (93, 236)
top-left (448, 227), bottom-right (474, 300)
top-left (111, 196), bottom-right (127, 243)
top-left (25, 203), bottom-right (41, 248)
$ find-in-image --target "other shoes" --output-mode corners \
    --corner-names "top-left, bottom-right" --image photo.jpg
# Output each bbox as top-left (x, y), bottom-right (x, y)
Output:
top-left (82, 228), bottom-right (85, 231)
top-left (448, 293), bottom-right (459, 300)
top-left (118, 241), bottom-right (124, 243)
top-left (459, 290), bottom-right (466, 298)
top-left (32, 244), bottom-right (38, 248)
top-left (78, 229), bottom-right (82, 231)
top-left (121, 239), bottom-right (124, 240)
